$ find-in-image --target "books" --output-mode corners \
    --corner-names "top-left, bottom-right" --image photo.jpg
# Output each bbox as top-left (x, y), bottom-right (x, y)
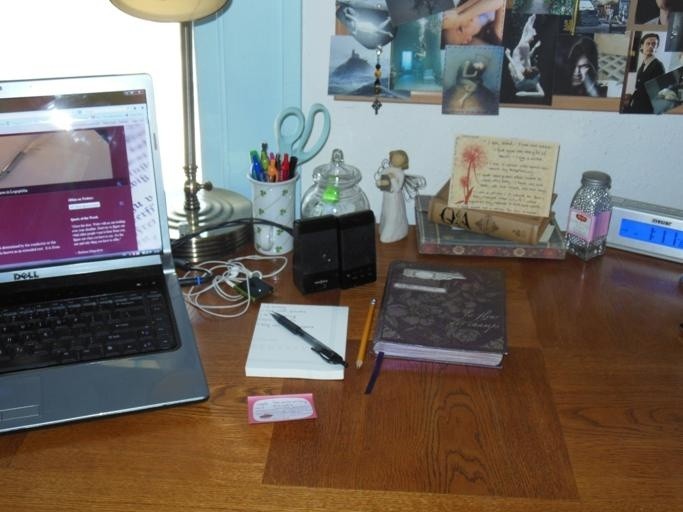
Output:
top-left (244, 302), bottom-right (349, 381)
top-left (415, 177), bottom-right (567, 261)
top-left (371, 260), bottom-right (509, 370)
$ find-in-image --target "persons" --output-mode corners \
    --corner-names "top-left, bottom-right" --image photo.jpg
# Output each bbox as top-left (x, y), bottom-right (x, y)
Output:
top-left (386, 0), bottom-right (507, 45)
top-left (643, 0), bottom-right (682, 52)
top-left (376, 151), bottom-right (426, 243)
top-left (658, 77), bottom-right (682, 114)
top-left (555, 38), bottom-right (607, 98)
top-left (444, 63), bottom-right (497, 112)
top-left (629, 34), bottom-right (666, 114)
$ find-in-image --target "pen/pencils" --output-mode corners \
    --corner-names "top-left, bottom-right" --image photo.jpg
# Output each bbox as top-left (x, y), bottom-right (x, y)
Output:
top-left (179, 275), bottom-right (210, 285)
top-left (222, 277), bottom-right (256, 302)
top-left (270, 311), bottom-right (349, 369)
top-left (355, 297), bottom-right (377, 369)
top-left (250, 142), bottom-right (298, 182)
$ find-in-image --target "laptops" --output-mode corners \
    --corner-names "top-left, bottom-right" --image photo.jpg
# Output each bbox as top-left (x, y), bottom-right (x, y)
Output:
top-left (0, 72), bottom-right (211, 435)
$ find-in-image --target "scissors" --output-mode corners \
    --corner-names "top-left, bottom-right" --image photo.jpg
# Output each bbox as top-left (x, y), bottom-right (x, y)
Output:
top-left (274, 103), bottom-right (331, 166)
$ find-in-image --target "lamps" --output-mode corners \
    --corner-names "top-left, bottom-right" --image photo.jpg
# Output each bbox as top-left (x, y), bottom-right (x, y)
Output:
top-left (113, 2), bottom-right (254, 263)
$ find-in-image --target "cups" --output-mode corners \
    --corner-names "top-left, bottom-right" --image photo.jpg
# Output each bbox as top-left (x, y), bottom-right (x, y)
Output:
top-left (247, 173), bottom-right (298, 257)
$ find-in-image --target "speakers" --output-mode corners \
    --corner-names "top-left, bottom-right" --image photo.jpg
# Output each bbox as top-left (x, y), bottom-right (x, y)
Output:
top-left (338, 210), bottom-right (377, 290)
top-left (292, 213), bottom-right (340, 295)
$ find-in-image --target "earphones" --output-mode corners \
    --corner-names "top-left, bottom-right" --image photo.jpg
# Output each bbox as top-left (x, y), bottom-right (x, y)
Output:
top-left (230, 269), bottom-right (240, 276)
top-left (251, 270), bottom-right (262, 280)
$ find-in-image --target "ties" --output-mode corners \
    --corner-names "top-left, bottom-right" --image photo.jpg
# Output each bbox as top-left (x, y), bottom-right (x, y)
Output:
top-left (635, 63), bottom-right (645, 89)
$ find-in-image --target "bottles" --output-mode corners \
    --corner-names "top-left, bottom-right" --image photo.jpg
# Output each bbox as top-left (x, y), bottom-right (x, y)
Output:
top-left (300, 148), bottom-right (369, 220)
top-left (564, 169), bottom-right (614, 262)
